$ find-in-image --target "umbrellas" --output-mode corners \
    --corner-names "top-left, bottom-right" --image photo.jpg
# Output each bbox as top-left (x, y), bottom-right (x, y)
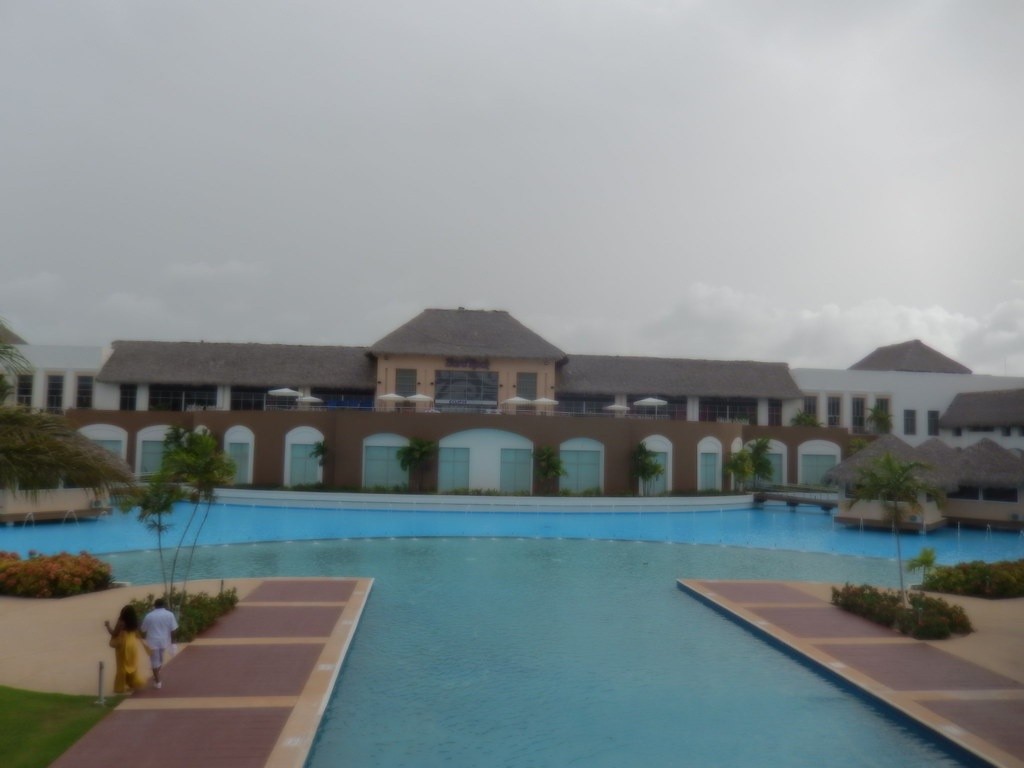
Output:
top-left (406, 394), bottom-right (434, 402)
top-left (602, 405), bottom-right (630, 410)
top-left (501, 397), bottom-right (531, 405)
top-left (268, 388), bottom-right (303, 397)
top-left (377, 393), bottom-right (406, 401)
top-left (296, 395), bottom-right (324, 402)
top-left (531, 398), bottom-right (560, 406)
top-left (633, 397), bottom-right (667, 420)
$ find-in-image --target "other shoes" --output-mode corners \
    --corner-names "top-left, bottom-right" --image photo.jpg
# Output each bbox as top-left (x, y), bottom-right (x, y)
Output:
top-left (154, 681), bottom-right (162, 689)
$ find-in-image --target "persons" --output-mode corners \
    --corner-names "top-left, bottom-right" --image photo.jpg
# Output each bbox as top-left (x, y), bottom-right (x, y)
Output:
top-left (139, 599), bottom-right (179, 690)
top-left (104, 605), bottom-right (154, 694)
